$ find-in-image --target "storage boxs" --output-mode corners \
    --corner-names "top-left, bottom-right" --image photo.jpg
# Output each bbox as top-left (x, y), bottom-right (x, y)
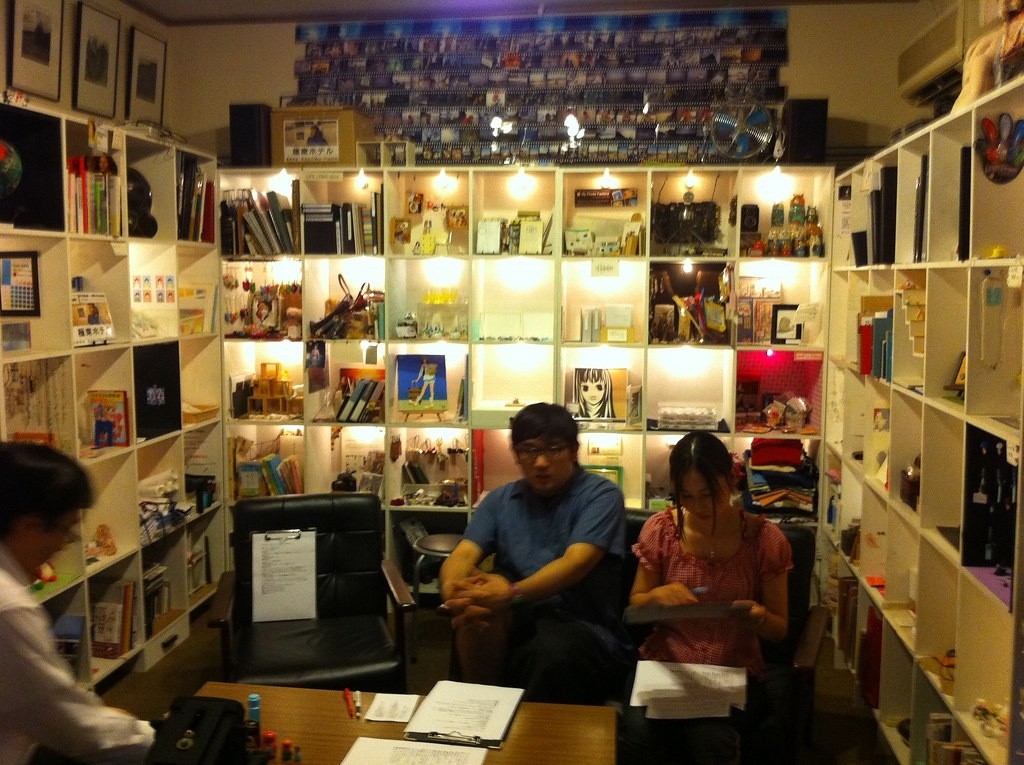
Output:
top-left (268, 107), bottom-right (374, 165)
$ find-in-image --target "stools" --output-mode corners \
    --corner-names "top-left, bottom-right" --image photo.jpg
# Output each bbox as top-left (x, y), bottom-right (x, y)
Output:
top-left (409, 531), bottom-right (462, 665)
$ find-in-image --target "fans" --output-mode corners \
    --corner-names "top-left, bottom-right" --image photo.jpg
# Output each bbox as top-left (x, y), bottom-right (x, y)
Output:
top-left (709, 95), bottom-right (775, 163)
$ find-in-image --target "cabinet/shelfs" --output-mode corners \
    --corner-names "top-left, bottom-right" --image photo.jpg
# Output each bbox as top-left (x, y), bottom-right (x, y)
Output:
top-left (820, 74), bottom-right (1024, 762)
top-left (208, 165), bottom-right (837, 603)
top-left (0, 91), bottom-right (221, 692)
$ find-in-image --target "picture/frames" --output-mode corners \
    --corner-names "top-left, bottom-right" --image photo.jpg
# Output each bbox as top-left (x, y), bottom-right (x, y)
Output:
top-left (128, 22), bottom-right (167, 123)
top-left (72, 0), bottom-right (122, 118)
top-left (7, 0), bottom-right (65, 102)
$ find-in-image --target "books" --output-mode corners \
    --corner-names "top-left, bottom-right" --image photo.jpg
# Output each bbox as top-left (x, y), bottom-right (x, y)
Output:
top-left (913, 144), bottom-right (969, 263)
top-left (302, 184), bottom-right (383, 254)
top-left (91, 566), bottom-right (172, 656)
top-left (305, 125), bottom-right (326, 147)
top-left (923, 712), bottom-right (986, 765)
top-left (338, 378), bottom-right (385, 422)
top-left (178, 150), bottom-right (214, 243)
top-left (837, 159), bottom-right (893, 262)
top-left (857, 295), bottom-right (893, 381)
top-left (223, 188), bottom-right (293, 256)
top-left (66, 154), bottom-right (121, 237)
top-left (227, 435), bottom-right (303, 496)
top-left (85, 389), bottom-right (130, 448)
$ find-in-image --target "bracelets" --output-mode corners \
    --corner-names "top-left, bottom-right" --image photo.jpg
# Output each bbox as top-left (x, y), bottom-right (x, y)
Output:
top-left (510, 583), bottom-right (523, 603)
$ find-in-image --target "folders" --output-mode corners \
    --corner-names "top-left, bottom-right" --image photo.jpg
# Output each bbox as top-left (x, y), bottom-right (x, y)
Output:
top-left (358, 380), bottom-right (385, 422)
top-left (349, 380), bottom-right (378, 423)
top-left (339, 379), bottom-right (371, 422)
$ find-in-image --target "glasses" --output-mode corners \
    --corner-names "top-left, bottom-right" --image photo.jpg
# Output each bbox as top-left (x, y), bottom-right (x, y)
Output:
top-left (513, 445), bottom-right (572, 461)
top-left (35, 514), bottom-right (78, 546)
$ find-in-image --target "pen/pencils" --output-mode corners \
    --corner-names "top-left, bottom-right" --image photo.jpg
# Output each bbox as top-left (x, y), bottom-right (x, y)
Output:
top-left (344, 688), bottom-right (354, 718)
top-left (691, 587), bottom-right (707, 595)
top-left (354, 691), bottom-right (362, 719)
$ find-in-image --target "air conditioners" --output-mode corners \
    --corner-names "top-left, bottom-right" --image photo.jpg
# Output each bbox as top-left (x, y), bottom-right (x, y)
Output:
top-left (896, 2), bottom-right (983, 107)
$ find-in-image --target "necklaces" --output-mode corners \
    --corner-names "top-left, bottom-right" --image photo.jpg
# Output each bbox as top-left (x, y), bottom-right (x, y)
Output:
top-left (710, 547), bottom-right (714, 558)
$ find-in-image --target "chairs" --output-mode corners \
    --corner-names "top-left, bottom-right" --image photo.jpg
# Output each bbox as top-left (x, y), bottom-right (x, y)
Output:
top-left (626, 504), bottom-right (816, 763)
top-left (207, 491), bottom-right (415, 695)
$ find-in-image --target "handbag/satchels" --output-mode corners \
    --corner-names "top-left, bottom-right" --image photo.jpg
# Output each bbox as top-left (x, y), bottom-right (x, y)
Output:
top-left (144, 695), bottom-right (246, 765)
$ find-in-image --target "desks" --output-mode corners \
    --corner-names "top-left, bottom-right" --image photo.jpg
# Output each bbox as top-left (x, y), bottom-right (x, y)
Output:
top-left (193, 680), bottom-right (618, 764)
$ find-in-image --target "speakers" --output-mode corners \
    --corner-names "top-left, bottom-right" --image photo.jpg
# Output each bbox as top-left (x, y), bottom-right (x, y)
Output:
top-left (780, 98), bottom-right (829, 161)
top-left (229, 103), bottom-right (271, 168)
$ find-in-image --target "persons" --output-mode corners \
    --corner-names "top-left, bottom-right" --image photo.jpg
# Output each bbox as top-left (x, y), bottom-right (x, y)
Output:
top-left (87, 303), bottom-right (101, 323)
top-left (0, 441), bottom-right (158, 765)
top-left (93, 152), bottom-right (117, 175)
top-left (617, 432), bottom-right (793, 765)
top-left (439, 403), bottom-right (625, 703)
